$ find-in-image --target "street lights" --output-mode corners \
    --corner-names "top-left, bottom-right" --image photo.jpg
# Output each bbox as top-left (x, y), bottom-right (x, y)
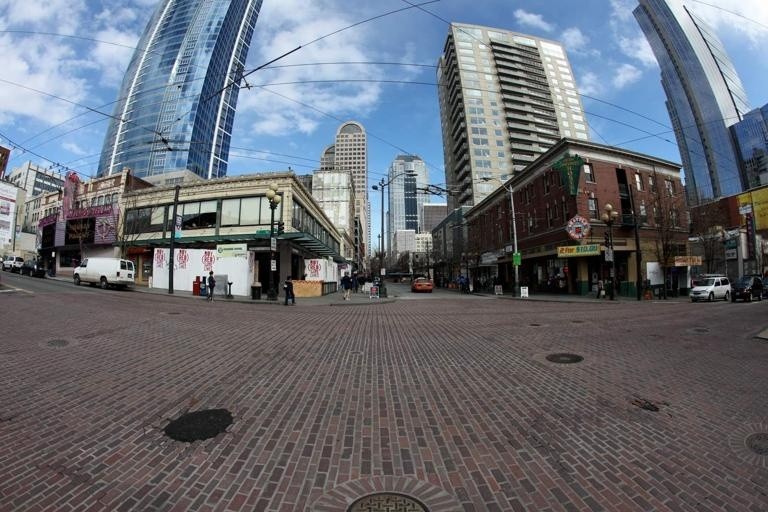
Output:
top-left (377, 172), bottom-right (417, 288)
top-left (265, 181), bottom-right (282, 300)
top-left (482, 176), bottom-right (521, 297)
top-left (600, 203), bottom-right (620, 300)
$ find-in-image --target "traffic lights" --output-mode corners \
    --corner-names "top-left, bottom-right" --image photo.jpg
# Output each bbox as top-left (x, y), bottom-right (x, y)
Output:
top-left (603, 231), bottom-right (612, 249)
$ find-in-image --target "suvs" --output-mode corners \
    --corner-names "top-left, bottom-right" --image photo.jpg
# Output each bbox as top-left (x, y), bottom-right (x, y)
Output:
top-left (2, 256), bottom-right (25, 272)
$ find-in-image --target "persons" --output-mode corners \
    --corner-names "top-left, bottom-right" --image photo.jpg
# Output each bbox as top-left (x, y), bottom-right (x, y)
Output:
top-left (284, 276), bottom-right (296, 305)
top-left (597, 280), bottom-right (606, 299)
top-left (341, 271), bottom-right (359, 300)
top-left (208, 271), bottom-right (216, 301)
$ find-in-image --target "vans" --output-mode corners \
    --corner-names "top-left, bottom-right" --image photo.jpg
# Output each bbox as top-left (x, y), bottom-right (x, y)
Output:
top-left (73, 257), bottom-right (138, 292)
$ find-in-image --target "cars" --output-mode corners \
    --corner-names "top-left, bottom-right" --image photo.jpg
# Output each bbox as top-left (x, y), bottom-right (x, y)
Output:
top-left (409, 277), bottom-right (433, 293)
top-left (20, 259), bottom-right (47, 278)
top-left (690, 275), bottom-right (768, 305)
